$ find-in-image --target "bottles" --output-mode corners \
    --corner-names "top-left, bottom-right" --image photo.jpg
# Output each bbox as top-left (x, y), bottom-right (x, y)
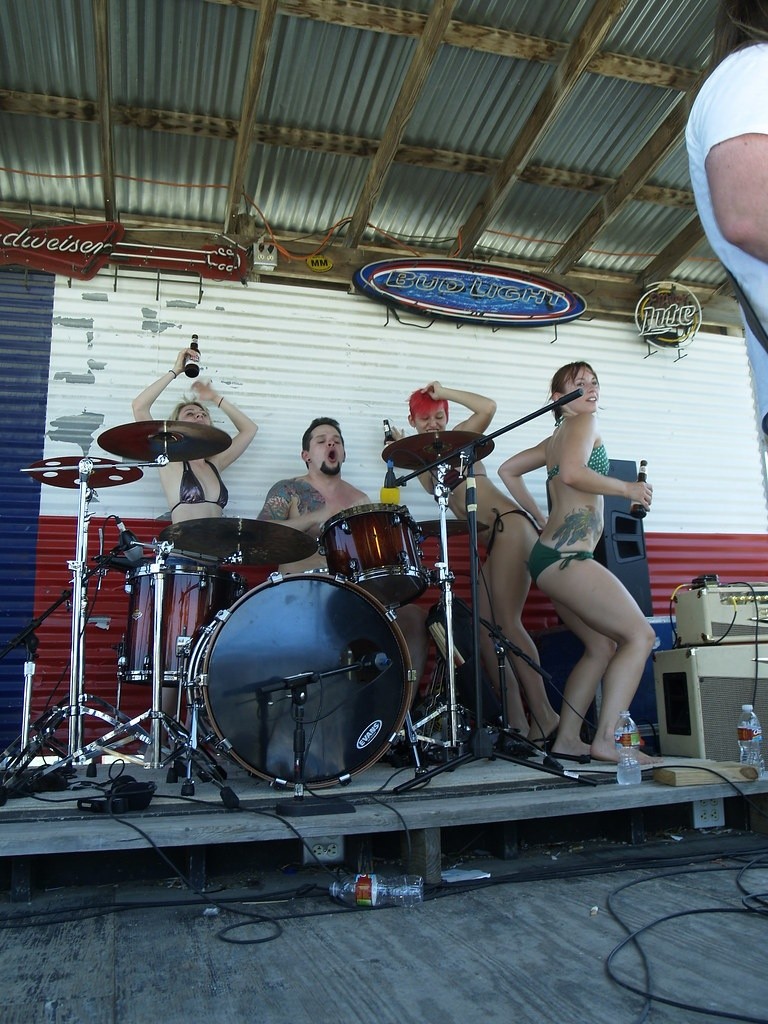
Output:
top-left (383, 420), bottom-right (396, 448)
top-left (185, 333), bottom-right (202, 378)
top-left (738, 704), bottom-right (765, 778)
top-left (631, 460), bottom-right (649, 518)
top-left (614, 710), bottom-right (641, 785)
top-left (328, 872), bottom-right (424, 908)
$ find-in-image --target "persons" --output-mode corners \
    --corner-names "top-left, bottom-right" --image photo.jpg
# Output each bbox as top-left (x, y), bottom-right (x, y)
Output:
top-left (498, 362), bottom-right (659, 765)
top-left (132, 347), bottom-right (259, 763)
top-left (256, 417), bottom-right (427, 713)
top-left (391, 380), bottom-right (560, 750)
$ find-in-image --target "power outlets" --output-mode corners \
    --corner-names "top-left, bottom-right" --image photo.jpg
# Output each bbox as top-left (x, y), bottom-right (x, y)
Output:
top-left (693, 797), bottom-right (724, 828)
top-left (304, 837), bottom-right (344, 865)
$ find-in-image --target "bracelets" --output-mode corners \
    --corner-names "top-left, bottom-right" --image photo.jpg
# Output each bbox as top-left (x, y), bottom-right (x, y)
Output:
top-left (217, 396), bottom-right (224, 409)
top-left (168, 370), bottom-right (177, 379)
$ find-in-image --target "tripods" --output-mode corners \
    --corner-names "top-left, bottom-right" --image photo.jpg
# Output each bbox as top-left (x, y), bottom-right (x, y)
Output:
top-left (0, 433), bottom-right (228, 807)
top-left (391, 388), bottom-right (599, 794)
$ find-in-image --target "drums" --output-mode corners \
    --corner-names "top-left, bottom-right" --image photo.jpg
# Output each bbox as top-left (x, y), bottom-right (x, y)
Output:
top-left (321, 503), bottom-right (430, 610)
top-left (120, 559), bottom-right (250, 688)
top-left (184, 572), bottom-right (418, 790)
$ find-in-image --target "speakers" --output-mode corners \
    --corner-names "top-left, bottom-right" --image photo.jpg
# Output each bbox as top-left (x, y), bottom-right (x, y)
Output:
top-left (546, 459), bottom-right (654, 625)
top-left (653, 642), bottom-right (768, 771)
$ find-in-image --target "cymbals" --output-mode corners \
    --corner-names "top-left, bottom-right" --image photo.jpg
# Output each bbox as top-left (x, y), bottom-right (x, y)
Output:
top-left (382, 430), bottom-right (495, 470)
top-left (27, 456), bottom-right (143, 489)
top-left (96, 420), bottom-right (233, 463)
top-left (160, 517), bottom-right (320, 565)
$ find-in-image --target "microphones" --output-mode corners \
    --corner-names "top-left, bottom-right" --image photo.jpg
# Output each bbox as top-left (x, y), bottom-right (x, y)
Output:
top-left (114, 516), bottom-right (143, 561)
top-left (380, 460), bottom-right (400, 506)
top-left (360, 651), bottom-right (390, 670)
